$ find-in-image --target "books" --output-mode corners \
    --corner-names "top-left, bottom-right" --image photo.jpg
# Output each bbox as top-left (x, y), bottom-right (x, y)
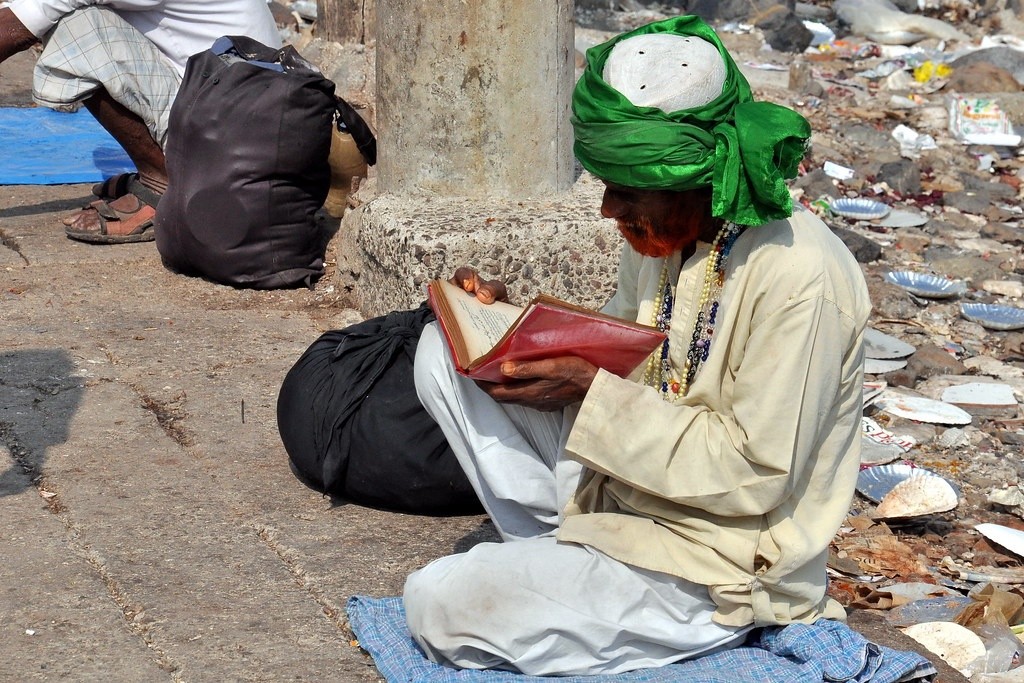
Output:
top-left (426, 275), bottom-right (671, 385)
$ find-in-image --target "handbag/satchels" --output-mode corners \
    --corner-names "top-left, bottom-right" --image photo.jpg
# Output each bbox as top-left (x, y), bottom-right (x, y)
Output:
top-left (155, 34), bottom-right (378, 293)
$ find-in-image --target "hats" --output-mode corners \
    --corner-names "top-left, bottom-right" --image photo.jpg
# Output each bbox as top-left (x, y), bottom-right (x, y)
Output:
top-left (602, 32), bottom-right (725, 111)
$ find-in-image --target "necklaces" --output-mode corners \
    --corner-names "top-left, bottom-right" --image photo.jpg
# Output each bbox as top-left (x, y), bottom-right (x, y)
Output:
top-left (640, 221), bottom-right (746, 403)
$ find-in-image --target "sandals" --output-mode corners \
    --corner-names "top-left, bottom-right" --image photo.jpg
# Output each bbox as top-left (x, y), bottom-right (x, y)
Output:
top-left (62, 178), bottom-right (162, 244)
top-left (90, 171), bottom-right (140, 201)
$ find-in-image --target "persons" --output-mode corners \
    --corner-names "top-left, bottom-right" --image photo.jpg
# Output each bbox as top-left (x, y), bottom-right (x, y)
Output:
top-left (0, 0), bottom-right (284, 245)
top-left (402, 14), bottom-right (873, 677)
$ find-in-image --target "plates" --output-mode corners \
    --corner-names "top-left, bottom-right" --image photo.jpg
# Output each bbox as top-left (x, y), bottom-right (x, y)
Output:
top-left (873, 397), bottom-right (973, 425)
top-left (884, 596), bottom-right (975, 631)
top-left (884, 269), bottom-right (962, 298)
top-left (863, 327), bottom-right (916, 359)
top-left (864, 356), bottom-right (907, 374)
top-left (856, 462), bottom-right (961, 513)
top-left (800, 20), bottom-right (837, 47)
top-left (941, 383), bottom-right (1019, 406)
top-left (830, 197), bottom-right (893, 220)
top-left (959, 302), bottom-right (1024, 329)
top-left (899, 621), bottom-right (987, 680)
top-left (873, 208), bottom-right (931, 229)
